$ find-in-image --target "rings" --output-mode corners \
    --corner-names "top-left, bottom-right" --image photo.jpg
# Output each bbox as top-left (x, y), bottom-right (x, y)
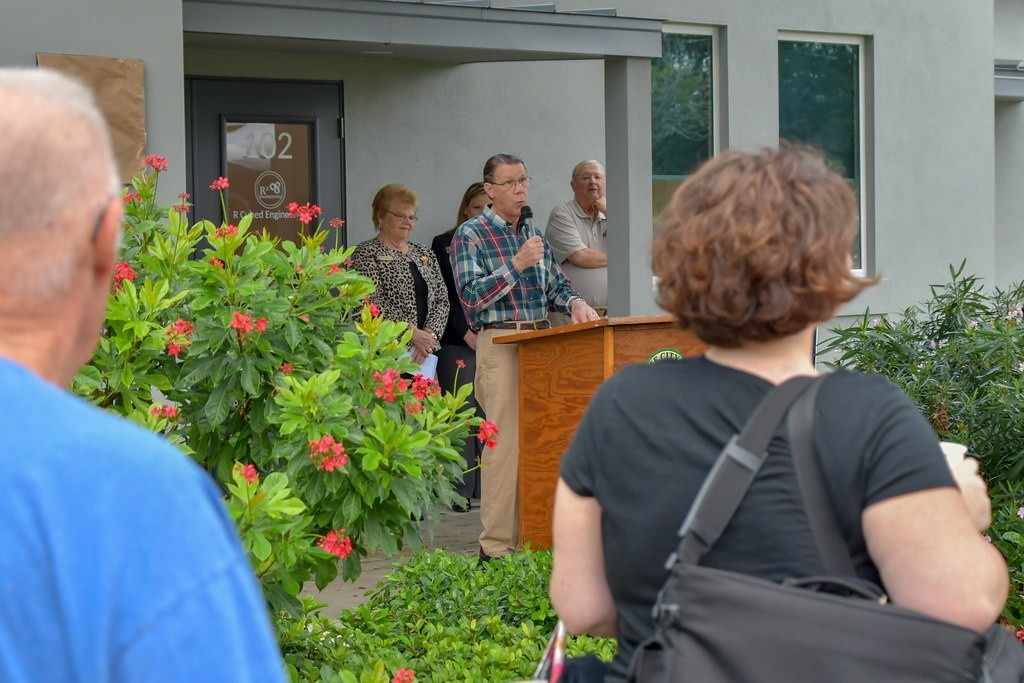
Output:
top-left (593, 314), bottom-right (597, 317)
top-left (428, 348), bottom-right (431, 352)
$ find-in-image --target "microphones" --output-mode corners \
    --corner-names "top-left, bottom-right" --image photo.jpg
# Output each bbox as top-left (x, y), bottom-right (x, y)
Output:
top-left (521, 205), bottom-right (535, 241)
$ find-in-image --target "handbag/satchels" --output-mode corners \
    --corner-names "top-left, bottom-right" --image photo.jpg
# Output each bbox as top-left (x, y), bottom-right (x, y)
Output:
top-left (628, 374), bottom-right (1024, 683)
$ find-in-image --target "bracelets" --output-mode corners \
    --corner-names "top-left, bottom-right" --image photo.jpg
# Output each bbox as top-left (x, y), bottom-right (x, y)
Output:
top-left (426, 329), bottom-right (437, 340)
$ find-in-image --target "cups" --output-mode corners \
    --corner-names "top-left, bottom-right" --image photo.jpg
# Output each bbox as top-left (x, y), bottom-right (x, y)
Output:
top-left (938, 441), bottom-right (966, 466)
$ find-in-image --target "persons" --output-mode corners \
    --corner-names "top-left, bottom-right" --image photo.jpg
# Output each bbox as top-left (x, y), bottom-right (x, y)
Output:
top-left (0, 67), bottom-right (285, 683)
top-left (544, 159), bottom-right (608, 328)
top-left (348, 184), bottom-right (450, 521)
top-left (549, 148), bottom-right (1009, 683)
top-left (430, 182), bottom-right (491, 513)
top-left (449, 153), bottom-right (601, 567)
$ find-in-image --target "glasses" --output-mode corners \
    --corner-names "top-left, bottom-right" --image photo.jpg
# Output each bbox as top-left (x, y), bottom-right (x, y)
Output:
top-left (487, 175), bottom-right (532, 188)
top-left (383, 208), bottom-right (418, 224)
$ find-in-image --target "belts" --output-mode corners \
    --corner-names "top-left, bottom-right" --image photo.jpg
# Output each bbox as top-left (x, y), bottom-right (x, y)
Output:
top-left (594, 310), bottom-right (608, 316)
top-left (477, 319), bottom-right (550, 331)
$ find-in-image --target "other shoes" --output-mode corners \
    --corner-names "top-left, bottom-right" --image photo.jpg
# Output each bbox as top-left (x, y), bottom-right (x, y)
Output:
top-left (410, 512), bottom-right (424, 521)
top-left (454, 497), bottom-right (471, 512)
